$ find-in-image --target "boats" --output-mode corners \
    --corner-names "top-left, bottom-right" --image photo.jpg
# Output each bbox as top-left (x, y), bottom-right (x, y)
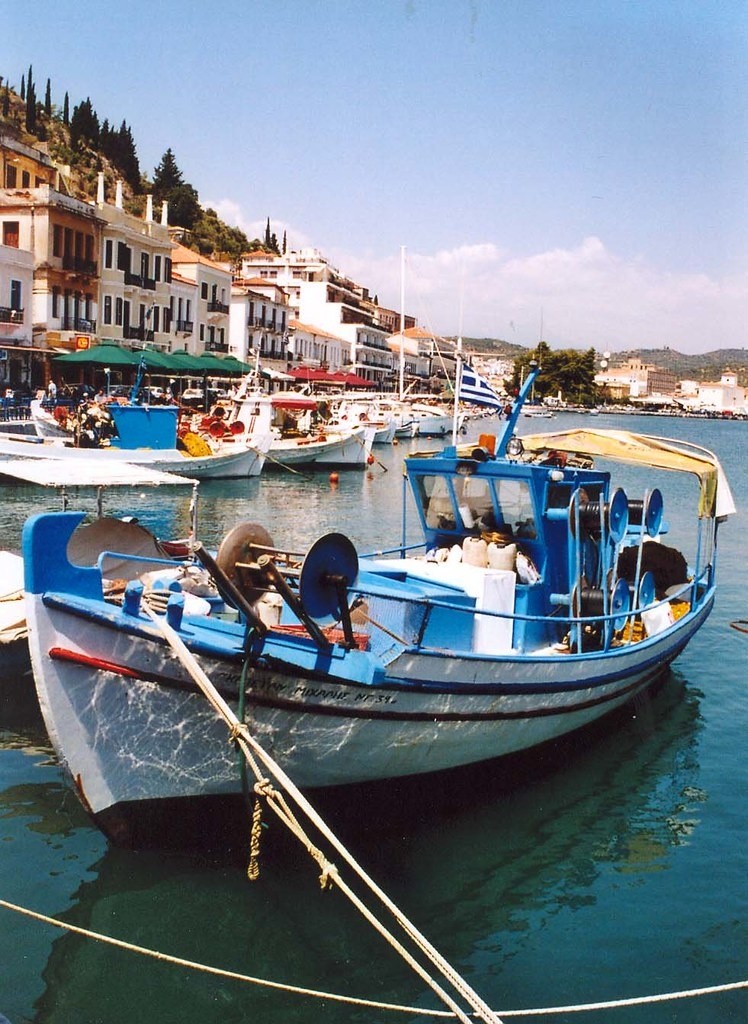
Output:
top-left (4, 327), bottom-right (467, 480)
top-left (23, 369), bottom-right (738, 855)
top-left (525, 409), bottom-right (555, 419)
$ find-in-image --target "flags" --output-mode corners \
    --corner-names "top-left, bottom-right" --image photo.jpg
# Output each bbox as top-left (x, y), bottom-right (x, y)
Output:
top-left (459, 363), bottom-right (503, 420)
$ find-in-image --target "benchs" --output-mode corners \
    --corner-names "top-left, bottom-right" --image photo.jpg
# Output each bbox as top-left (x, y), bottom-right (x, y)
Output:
top-left (425, 530), bottom-right (553, 652)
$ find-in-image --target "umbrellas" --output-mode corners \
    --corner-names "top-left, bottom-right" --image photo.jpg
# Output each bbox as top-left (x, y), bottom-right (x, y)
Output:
top-left (51, 340), bottom-right (375, 388)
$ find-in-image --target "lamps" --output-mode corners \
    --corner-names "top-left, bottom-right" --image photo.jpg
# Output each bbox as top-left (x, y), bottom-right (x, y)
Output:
top-left (504, 437), bottom-right (525, 464)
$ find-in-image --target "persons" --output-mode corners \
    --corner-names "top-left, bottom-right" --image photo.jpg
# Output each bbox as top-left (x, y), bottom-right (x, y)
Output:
top-left (48, 379), bottom-right (105, 440)
